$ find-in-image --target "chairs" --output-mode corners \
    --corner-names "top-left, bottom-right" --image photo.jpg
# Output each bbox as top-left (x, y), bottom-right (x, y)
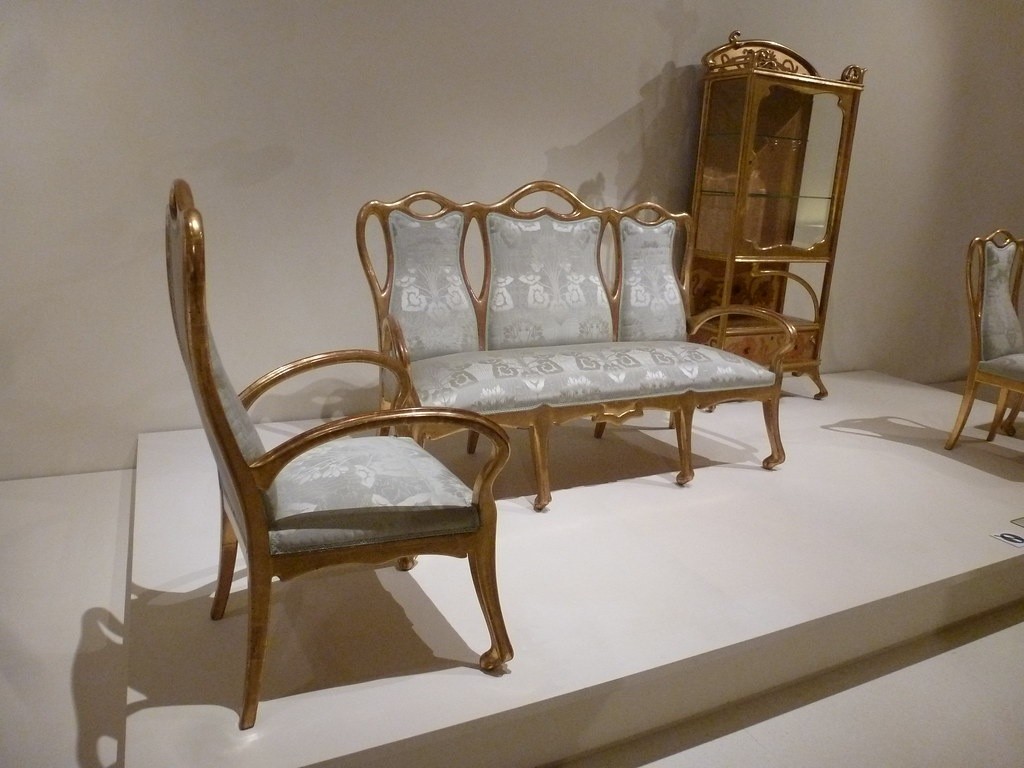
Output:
top-left (165, 178), bottom-right (514, 731)
top-left (944, 228), bottom-right (1024, 451)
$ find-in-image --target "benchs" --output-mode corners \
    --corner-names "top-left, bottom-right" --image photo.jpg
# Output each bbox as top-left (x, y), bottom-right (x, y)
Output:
top-left (356, 180), bottom-right (797, 514)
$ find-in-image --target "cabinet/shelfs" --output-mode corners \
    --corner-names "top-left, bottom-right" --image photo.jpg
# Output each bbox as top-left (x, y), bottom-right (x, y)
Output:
top-left (682, 31), bottom-right (868, 400)
